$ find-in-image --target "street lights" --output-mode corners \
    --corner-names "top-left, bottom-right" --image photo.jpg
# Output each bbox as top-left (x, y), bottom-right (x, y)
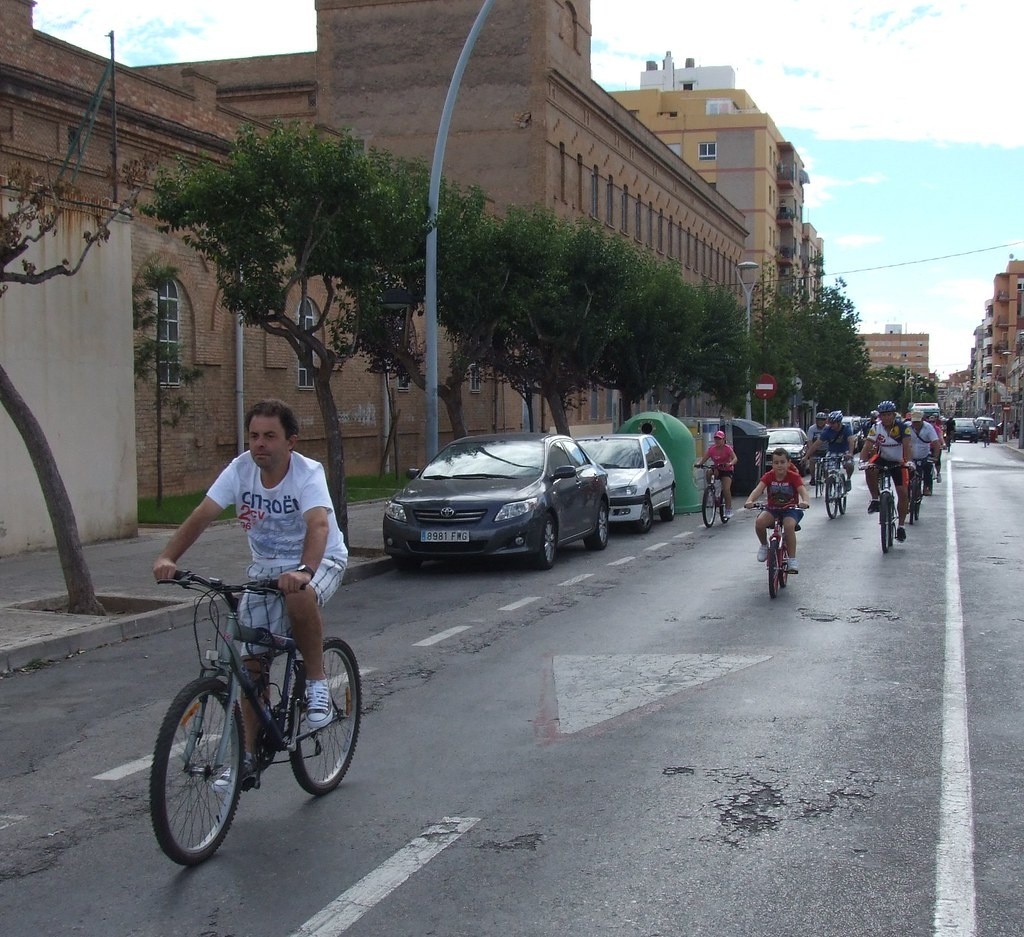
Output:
top-left (1000, 351), bottom-right (1013, 442)
top-left (735, 261), bottom-right (759, 420)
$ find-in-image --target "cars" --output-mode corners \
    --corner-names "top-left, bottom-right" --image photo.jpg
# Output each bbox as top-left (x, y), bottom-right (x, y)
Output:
top-left (760, 425), bottom-right (809, 477)
top-left (973, 417), bottom-right (996, 441)
top-left (951, 417), bottom-right (978, 444)
top-left (382, 432), bottom-right (611, 570)
top-left (838, 402), bottom-right (949, 452)
top-left (731, 418), bottom-right (768, 437)
top-left (570, 434), bottom-right (677, 531)
top-left (997, 420), bottom-right (1015, 435)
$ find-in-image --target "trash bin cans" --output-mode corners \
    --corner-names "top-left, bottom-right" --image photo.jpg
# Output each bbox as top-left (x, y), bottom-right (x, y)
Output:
top-left (719, 419), bottom-right (770, 496)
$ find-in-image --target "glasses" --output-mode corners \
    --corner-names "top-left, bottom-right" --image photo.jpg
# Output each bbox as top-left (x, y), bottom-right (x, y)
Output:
top-left (929, 421), bottom-right (936, 423)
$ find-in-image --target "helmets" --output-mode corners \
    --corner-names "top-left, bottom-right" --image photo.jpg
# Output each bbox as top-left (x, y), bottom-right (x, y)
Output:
top-left (827, 411), bottom-right (843, 421)
top-left (878, 401), bottom-right (896, 413)
top-left (870, 411), bottom-right (880, 419)
top-left (815, 412), bottom-right (827, 419)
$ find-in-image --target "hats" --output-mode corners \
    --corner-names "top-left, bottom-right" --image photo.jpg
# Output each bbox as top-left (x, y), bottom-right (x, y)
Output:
top-left (911, 409), bottom-right (924, 421)
top-left (714, 431), bottom-right (725, 438)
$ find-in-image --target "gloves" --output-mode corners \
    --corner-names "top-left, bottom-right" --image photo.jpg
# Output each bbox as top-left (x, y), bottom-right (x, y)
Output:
top-left (904, 461), bottom-right (916, 470)
top-left (858, 460), bottom-right (868, 470)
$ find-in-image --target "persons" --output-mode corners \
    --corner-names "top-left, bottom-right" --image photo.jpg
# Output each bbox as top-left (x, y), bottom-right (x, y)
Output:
top-left (693, 430), bottom-right (737, 519)
top-left (861, 407), bottom-right (1021, 501)
top-left (804, 411), bottom-right (828, 487)
top-left (797, 410), bottom-right (854, 492)
top-left (154, 396), bottom-right (349, 796)
top-left (858, 399), bottom-right (913, 543)
top-left (743, 448), bottom-right (811, 576)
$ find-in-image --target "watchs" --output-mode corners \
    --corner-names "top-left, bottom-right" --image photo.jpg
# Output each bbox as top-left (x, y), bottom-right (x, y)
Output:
top-left (295, 562), bottom-right (315, 579)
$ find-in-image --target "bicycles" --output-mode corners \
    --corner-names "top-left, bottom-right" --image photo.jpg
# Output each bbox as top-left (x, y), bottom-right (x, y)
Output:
top-left (901, 431), bottom-right (956, 521)
top-left (143, 561), bottom-right (366, 870)
top-left (858, 462), bottom-right (910, 550)
top-left (808, 455), bottom-right (830, 495)
top-left (743, 499), bottom-right (812, 596)
top-left (695, 462), bottom-right (736, 528)
top-left (818, 453), bottom-right (853, 515)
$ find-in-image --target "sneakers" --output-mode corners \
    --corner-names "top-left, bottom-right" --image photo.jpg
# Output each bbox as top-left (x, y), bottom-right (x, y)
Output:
top-left (725, 508), bottom-right (733, 517)
top-left (306, 679), bottom-right (333, 727)
top-left (937, 473), bottom-right (941, 483)
top-left (211, 757), bottom-right (255, 792)
top-left (896, 528), bottom-right (907, 542)
top-left (786, 557), bottom-right (799, 572)
top-left (757, 544), bottom-right (770, 562)
top-left (867, 501), bottom-right (879, 514)
top-left (923, 485), bottom-right (932, 495)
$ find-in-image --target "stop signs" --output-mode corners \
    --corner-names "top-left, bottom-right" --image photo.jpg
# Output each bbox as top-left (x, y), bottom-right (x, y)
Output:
top-left (754, 374), bottom-right (778, 398)
top-left (1002, 404), bottom-right (1011, 412)
